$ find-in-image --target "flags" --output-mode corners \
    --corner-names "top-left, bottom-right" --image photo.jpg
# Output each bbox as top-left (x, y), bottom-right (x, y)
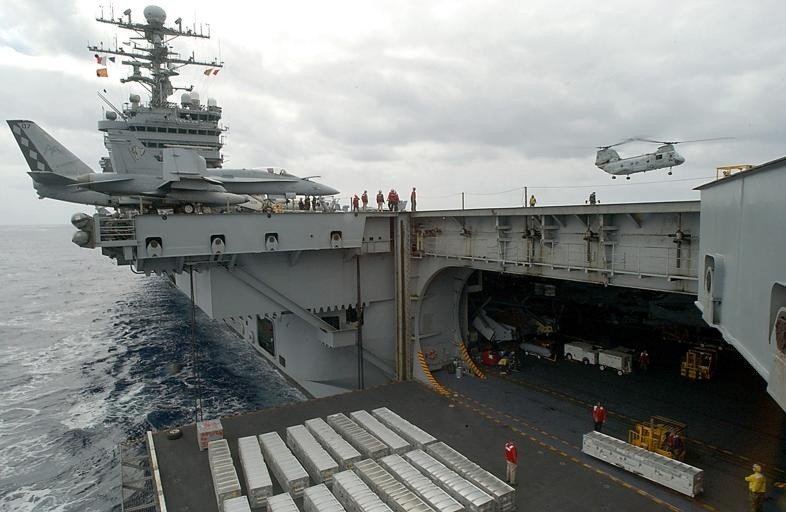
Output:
top-left (108, 57), bottom-right (115, 63)
top-left (96, 56), bottom-right (106, 65)
top-left (96, 68), bottom-right (108, 78)
top-left (204, 69), bottom-right (213, 76)
top-left (213, 70), bottom-right (220, 75)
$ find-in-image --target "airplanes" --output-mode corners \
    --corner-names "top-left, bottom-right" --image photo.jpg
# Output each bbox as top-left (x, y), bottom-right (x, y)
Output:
top-left (4, 119), bottom-right (340, 213)
top-left (594, 135), bottom-right (737, 179)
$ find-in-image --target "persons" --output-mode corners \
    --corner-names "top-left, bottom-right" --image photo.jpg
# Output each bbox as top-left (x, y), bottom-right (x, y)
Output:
top-left (411, 188), bottom-right (417, 212)
top-left (745, 463), bottom-right (767, 511)
top-left (589, 192), bottom-right (596, 205)
top-left (298, 197), bottom-right (304, 210)
top-left (377, 190), bottom-right (386, 212)
top-left (639, 350), bottom-right (650, 372)
top-left (387, 189), bottom-right (397, 212)
top-left (504, 438), bottom-right (518, 485)
top-left (353, 195), bottom-right (360, 212)
top-left (305, 196), bottom-right (310, 210)
top-left (361, 190), bottom-right (369, 212)
top-left (530, 195), bottom-right (537, 207)
top-left (393, 191), bottom-right (400, 211)
top-left (592, 401), bottom-right (607, 432)
top-left (311, 196), bottom-right (317, 211)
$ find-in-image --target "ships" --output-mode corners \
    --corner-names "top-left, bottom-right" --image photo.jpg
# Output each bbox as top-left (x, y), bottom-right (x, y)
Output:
top-left (71, 4), bottom-right (786, 512)
